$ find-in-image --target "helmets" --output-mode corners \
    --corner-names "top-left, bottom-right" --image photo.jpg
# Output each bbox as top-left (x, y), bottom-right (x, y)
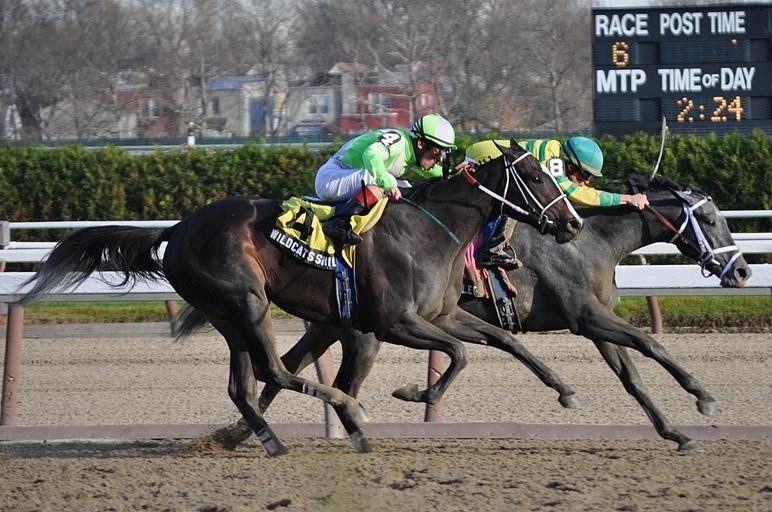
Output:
top-left (560, 137), bottom-right (604, 177)
top-left (408, 114), bottom-right (457, 151)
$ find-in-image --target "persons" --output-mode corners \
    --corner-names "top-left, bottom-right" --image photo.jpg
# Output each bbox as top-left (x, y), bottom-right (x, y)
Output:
top-left (186, 121), bottom-right (198, 145)
top-left (464, 136), bottom-right (649, 271)
top-left (315, 108), bottom-right (476, 247)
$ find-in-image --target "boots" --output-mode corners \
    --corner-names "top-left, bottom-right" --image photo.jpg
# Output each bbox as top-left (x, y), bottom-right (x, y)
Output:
top-left (322, 196), bottom-right (366, 244)
top-left (479, 216), bottom-right (523, 270)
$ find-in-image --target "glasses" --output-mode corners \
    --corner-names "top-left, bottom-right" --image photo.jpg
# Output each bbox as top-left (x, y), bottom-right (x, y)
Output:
top-left (561, 140), bottom-right (591, 185)
top-left (411, 116), bottom-right (446, 162)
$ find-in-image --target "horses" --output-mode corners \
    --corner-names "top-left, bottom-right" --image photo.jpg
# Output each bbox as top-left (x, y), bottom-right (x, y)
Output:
top-left (0, 136), bottom-right (586, 458)
top-left (167, 174), bottom-right (753, 451)
